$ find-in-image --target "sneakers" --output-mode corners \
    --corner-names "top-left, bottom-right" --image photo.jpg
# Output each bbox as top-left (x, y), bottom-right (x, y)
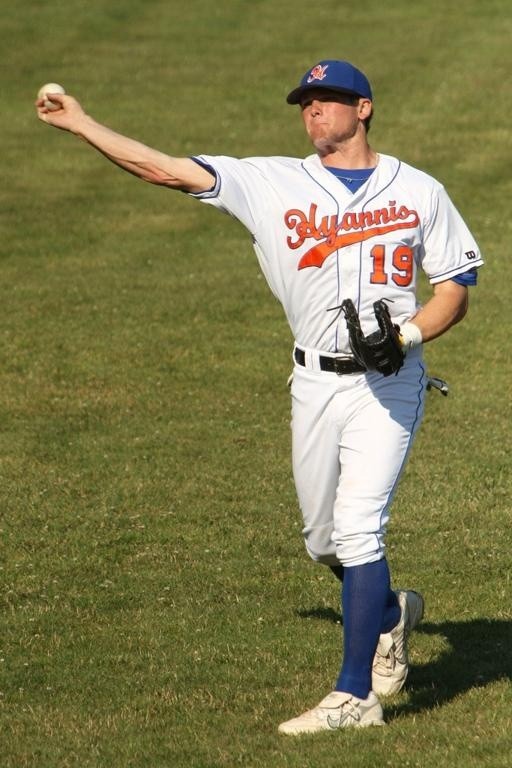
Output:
top-left (278, 690), bottom-right (386, 736)
top-left (371, 590), bottom-right (424, 697)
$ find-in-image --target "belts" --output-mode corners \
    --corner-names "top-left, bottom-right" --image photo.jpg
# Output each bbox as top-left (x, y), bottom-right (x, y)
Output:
top-left (295, 347), bottom-right (366, 375)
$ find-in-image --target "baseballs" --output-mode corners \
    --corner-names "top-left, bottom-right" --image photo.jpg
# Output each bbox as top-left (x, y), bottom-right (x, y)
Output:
top-left (37, 83), bottom-right (64, 111)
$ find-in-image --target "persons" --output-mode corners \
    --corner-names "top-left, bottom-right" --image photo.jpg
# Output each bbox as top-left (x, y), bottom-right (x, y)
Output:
top-left (36, 63), bottom-right (486, 734)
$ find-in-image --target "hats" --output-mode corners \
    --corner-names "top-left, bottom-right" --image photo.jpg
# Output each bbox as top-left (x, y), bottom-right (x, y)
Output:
top-left (287, 60), bottom-right (372, 104)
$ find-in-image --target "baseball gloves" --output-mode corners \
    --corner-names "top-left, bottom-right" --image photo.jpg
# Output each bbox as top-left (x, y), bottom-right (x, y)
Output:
top-left (326, 298), bottom-right (406, 377)
